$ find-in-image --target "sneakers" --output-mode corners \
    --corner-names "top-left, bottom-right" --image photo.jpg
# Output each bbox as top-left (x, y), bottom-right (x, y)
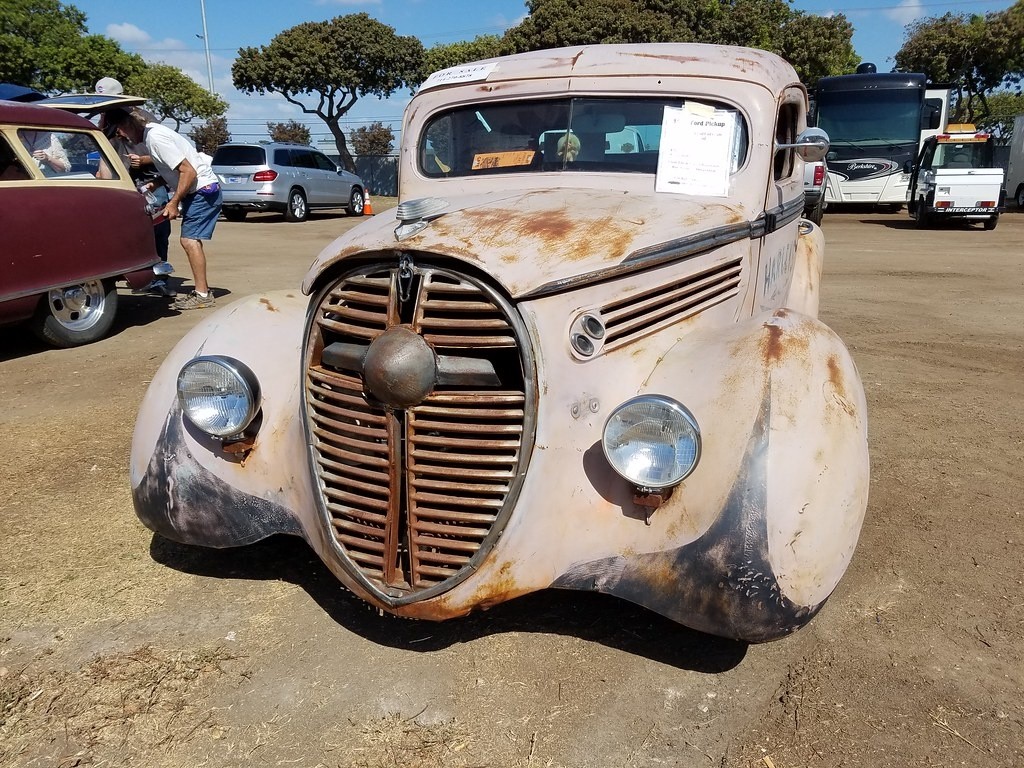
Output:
top-left (174, 290), bottom-right (216, 310)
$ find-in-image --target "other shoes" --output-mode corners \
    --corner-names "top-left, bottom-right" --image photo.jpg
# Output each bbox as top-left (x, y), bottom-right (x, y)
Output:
top-left (131, 272), bottom-right (169, 296)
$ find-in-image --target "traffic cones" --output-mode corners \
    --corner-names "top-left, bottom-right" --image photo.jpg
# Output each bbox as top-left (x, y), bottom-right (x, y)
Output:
top-left (362, 190), bottom-right (375, 218)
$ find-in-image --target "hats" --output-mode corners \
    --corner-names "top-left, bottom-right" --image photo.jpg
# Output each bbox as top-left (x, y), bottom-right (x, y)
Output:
top-left (104, 106), bottom-right (132, 141)
top-left (95, 77), bottom-right (123, 95)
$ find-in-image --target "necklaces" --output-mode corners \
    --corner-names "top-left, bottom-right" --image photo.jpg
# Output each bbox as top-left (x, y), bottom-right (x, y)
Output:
top-left (21, 130), bottom-right (37, 152)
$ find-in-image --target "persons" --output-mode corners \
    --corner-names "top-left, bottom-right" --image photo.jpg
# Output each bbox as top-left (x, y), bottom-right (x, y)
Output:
top-left (94, 77), bottom-right (222, 309)
top-left (17, 129), bottom-right (71, 177)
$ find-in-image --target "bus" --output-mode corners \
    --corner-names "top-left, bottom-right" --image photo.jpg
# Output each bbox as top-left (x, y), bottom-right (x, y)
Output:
top-left (807, 62), bottom-right (959, 215)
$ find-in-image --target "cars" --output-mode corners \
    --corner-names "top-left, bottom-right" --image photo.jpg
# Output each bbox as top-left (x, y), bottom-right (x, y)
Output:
top-left (1, 79), bottom-right (174, 345)
top-left (128, 41), bottom-right (871, 643)
top-left (209, 141), bottom-right (367, 223)
